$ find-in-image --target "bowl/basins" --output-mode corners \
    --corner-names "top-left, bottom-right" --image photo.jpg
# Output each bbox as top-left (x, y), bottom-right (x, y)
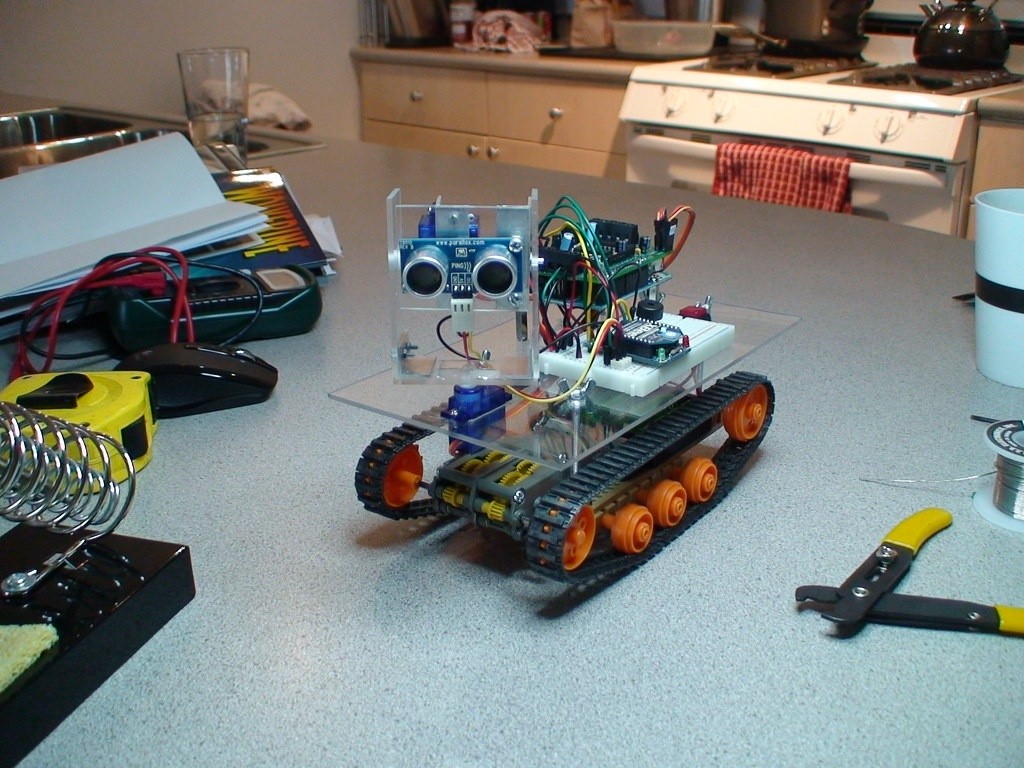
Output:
top-left (607, 19), bottom-right (716, 61)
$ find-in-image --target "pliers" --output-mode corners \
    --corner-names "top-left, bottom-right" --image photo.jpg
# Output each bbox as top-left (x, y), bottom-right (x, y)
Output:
top-left (795, 507), bottom-right (1024, 636)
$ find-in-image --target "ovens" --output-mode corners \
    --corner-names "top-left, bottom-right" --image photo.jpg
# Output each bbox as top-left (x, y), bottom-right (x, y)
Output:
top-left (624, 124), bottom-right (979, 239)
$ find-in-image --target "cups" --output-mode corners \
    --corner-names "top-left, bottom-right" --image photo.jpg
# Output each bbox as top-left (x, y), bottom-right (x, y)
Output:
top-left (176, 46), bottom-right (250, 167)
top-left (449, 2), bottom-right (477, 49)
top-left (974, 188), bottom-right (1024, 390)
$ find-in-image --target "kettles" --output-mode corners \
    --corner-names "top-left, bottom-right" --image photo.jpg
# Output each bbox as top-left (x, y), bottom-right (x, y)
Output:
top-left (912, 0), bottom-right (1010, 72)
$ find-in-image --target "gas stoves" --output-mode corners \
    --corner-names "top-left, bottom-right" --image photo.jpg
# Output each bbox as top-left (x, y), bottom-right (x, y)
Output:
top-left (617, 43), bottom-right (1024, 162)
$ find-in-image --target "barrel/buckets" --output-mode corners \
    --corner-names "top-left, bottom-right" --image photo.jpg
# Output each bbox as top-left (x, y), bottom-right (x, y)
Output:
top-left (971, 185), bottom-right (1024, 390)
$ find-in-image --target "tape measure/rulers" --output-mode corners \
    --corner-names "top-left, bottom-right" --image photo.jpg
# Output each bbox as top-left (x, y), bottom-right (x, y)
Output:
top-left (0, 370), bottom-right (156, 498)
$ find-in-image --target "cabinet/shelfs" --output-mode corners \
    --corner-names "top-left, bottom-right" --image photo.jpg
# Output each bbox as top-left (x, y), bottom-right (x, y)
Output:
top-left (964, 115), bottom-right (1024, 241)
top-left (348, 61), bottom-right (659, 183)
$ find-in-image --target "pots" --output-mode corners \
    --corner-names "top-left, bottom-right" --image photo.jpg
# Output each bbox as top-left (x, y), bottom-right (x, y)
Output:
top-left (711, 0), bottom-right (875, 60)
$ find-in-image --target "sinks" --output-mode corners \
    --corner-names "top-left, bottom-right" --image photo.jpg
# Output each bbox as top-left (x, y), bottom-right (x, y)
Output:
top-left (0, 103), bottom-right (137, 151)
top-left (0, 125), bottom-right (327, 184)
